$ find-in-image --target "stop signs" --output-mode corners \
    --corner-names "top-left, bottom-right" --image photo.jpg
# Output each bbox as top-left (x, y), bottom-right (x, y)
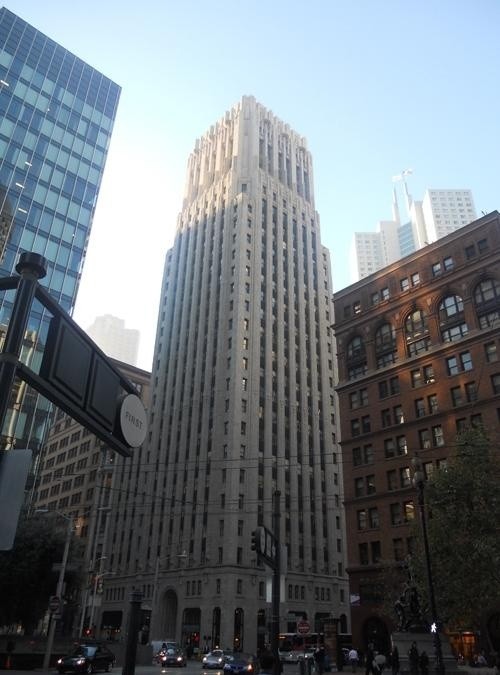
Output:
top-left (297, 621), bottom-right (311, 635)
top-left (48, 595), bottom-right (62, 614)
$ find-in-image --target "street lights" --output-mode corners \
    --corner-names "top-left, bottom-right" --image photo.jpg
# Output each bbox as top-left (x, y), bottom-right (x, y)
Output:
top-left (87, 571), bottom-right (117, 640)
top-left (34, 506), bottom-right (113, 671)
top-left (148, 553), bottom-right (188, 646)
top-left (410, 450), bottom-right (446, 674)
top-left (79, 555), bottom-right (108, 640)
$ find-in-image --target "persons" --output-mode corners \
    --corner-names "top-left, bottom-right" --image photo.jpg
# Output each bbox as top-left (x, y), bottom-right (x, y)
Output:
top-left (257, 639), bottom-right (500, 675)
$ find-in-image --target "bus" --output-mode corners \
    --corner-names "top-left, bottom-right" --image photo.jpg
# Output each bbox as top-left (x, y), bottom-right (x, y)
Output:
top-left (277, 632), bottom-right (353, 664)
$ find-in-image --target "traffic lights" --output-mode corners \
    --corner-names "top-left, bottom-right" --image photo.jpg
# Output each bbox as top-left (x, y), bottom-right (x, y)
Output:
top-left (85, 628), bottom-right (91, 635)
top-left (251, 532), bottom-right (256, 551)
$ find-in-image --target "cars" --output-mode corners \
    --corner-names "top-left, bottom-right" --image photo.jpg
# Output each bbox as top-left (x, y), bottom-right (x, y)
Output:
top-left (223, 652), bottom-right (260, 675)
top-left (56, 643), bottom-right (116, 675)
top-left (202, 650), bottom-right (225, 669)
top-left (162, 647), bottom-right (186, 667)
